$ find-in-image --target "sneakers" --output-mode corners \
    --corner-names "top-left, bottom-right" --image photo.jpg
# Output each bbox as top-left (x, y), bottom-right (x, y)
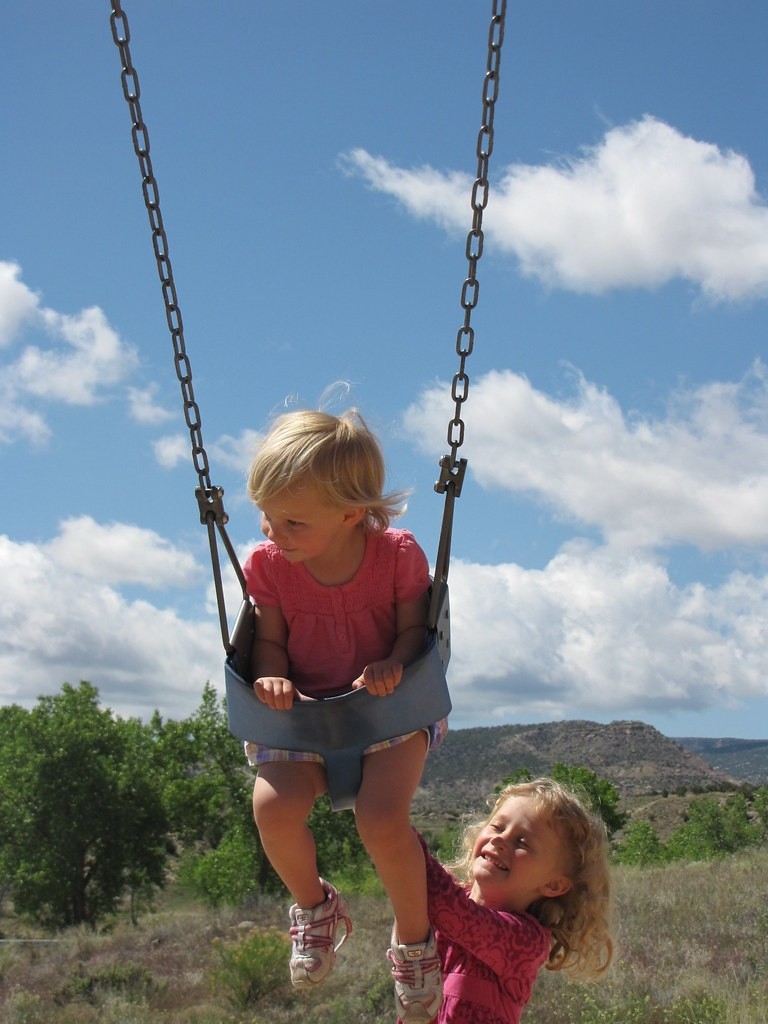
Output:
top-left (289, 878), bottom-right (352, 988)
top-left (385, 916), bottom-right (444, 1024)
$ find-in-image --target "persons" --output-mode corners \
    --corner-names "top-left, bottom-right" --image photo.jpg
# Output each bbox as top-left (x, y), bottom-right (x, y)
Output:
top-left (245, 409), bottom-right (448, 1023)
top-left (397, 778), bottom-right (614, 1024)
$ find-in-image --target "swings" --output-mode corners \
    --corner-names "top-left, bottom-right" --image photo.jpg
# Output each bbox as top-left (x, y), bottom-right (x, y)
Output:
top-left (111, 0), bottom-right (510, 811)
